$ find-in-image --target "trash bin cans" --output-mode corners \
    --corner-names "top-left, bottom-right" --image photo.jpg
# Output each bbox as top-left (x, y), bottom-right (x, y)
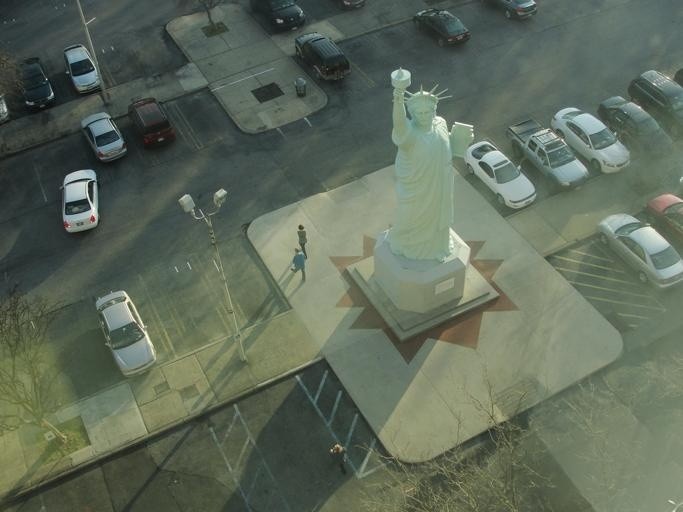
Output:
top-left (294, 78), bottom-right (306, 97)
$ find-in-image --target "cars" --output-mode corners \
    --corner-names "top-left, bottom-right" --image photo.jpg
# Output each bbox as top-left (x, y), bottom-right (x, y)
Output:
top-left (493, 0), bottom-right (541, 21)
top-left (331, 0), bottom-right (370, 11)
top-left (0, 88), bottom-right (11, 124)
top-left (17, 56), bottom-right (57, 113)
top-left (61, 43), bottom-right (103, 97)
top-left (57, 166), bottom-right (103, 240)
top-left (80, 111), bottom-right (129, 166)
top-left (125, 97), bottom-right (178, 151)
top-left (247, 0), bottom-right (310, 35)
top-left (94, 288), bottom-right (158, 381)
top-left (412, 6), bottom-right (473, 50)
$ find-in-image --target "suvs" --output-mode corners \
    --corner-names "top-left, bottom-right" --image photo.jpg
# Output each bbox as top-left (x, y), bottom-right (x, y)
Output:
top-left (291, 31), bottom-right (352, 84)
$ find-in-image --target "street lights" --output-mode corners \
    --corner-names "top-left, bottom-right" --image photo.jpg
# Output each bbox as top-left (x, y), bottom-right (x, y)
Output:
top-left (175, 185), bottom-right (249, 364)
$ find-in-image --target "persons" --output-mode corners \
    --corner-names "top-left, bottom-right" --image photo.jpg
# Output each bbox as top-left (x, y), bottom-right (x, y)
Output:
top-left (384, 64), bottom-right (474, 264)
top-left (296, 224), bottom-right (307, 258)
top-left (290, 247), bottom-right (306, 281)
